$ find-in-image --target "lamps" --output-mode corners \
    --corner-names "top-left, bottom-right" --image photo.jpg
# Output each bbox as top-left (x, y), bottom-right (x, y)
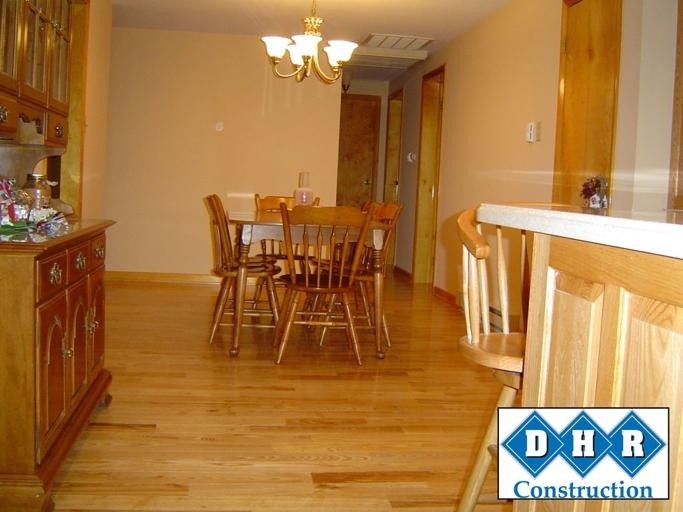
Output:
top-left (261, 1), bottom-right (359, 83)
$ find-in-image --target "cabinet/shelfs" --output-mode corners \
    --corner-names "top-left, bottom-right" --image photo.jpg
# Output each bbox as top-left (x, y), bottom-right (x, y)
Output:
top-left (0, 0), bottom-right (118, 512)
top-left (476, 202), bottom-right (682, 512)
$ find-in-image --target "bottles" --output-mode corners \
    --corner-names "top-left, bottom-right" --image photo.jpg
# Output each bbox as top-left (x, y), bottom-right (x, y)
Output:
top-left (295, 172), bottom-right (314, 206)
top-left (22, 173), bottom-right (51, 207)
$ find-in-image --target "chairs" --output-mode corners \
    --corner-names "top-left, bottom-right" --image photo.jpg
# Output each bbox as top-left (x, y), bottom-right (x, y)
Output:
top-left (456, 202), bottom-right (577, 512)
top-left (205, 193), bottom-right (404, 368)
